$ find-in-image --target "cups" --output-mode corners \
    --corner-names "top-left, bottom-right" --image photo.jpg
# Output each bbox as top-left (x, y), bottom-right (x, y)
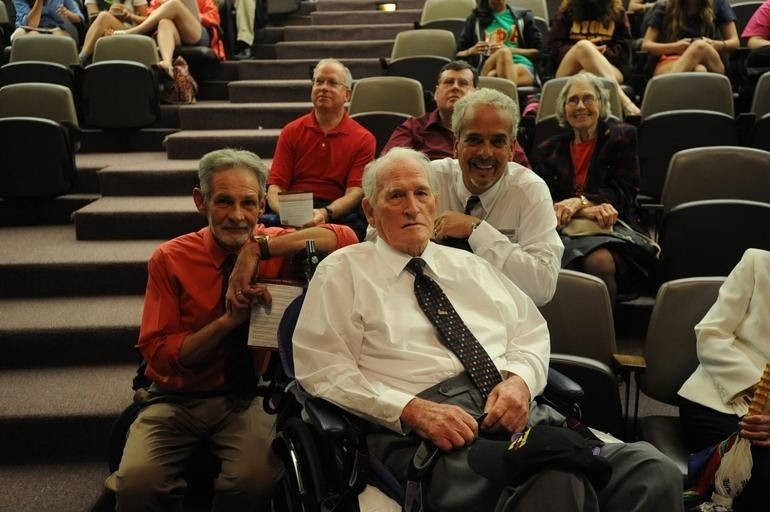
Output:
top-left (483, 32), bottom-right (495, 56)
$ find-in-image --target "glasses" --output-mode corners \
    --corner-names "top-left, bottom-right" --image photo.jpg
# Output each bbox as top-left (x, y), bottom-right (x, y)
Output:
top-left (564, 95), bottom-right (599, 105)
top-left (314, 78), bottom-right (349, 90)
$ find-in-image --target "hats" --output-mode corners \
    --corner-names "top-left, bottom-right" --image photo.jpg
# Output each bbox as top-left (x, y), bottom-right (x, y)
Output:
top-left (467, 424), bottom-right (598, 487)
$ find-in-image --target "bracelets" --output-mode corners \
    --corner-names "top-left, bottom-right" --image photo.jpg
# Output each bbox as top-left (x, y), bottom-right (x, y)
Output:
top-left (721, 39), bottom-right (727, 50)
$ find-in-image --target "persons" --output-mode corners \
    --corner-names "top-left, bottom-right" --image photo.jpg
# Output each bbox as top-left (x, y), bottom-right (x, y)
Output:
top-left (423, 89), bottom-right (563, 307)
top-left (105, 149), bottom-right (358, 512)
top-left (541, 0), bottom-right (642, 120)
top-left (0, 1), bottom-right (266, 105)
top-left (291, 146), bottom-right (683, 512)
top-left (634, 1), bottom-right (740, 79)
top-left (526, 72), bottom-right (639, 320)
top-left (740, 1), bottom-right (770, 57)
top-left (376, 60), bottom-right (531, 177)
top-left (456, 1), bottom-right (545, 89)
top-left (675, 247), bottom-right (769, 511)
top-left (257, 58), bottom-right (374, 244)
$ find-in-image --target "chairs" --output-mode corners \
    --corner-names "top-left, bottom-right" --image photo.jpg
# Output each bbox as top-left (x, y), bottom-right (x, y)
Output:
top-left (0, 31), bottom-right (80, 122)
top-left (414, 0), bottom-right (478, 42)
top-left (347, 77), bottom-right (426, 161)
top-left (636, 73), bottom-right (736, 203)
top-left (539, 267), bottom-right (622, 442)
top-left (535, 77), bottom-right (623, 163)
top-left (635, 278), bottom-right (737, 491)
top-left (0, 0), bottom-right (17, 53)
top-left (729, 0), bottom-right (765, 52)
top-left (739, 72), bottom-right (770, 158)
top-left (497, 1), bottom-right (550, 43)
top-left (0, 81), bottom-right (81, 200)
top-left (516, 85), bottom-right (540, 97)
top-left (473, 76), bottom-right (519, 105)
top-left (156, 44), bottom-right (219, 82)
top-left (658, 146), bottom-right (770, 282)
top-left (380, 29), bottom-right (457, 112)
top-left (264, 0), bottom-right (301, 17)
top-left (72, 32), bottom-right (160, 144)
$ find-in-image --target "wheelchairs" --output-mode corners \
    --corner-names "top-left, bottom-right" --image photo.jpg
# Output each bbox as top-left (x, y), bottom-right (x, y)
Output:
top-left (268, 291), bottom-right (583, 512)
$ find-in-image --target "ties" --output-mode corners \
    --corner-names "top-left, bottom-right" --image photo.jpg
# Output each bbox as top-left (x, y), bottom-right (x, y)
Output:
top-left (463, 196), bottom-right (481, 216)
top-left (404, 260), bottom-right (504, 406)
top-left (221, 251), bottom-right (261, 412)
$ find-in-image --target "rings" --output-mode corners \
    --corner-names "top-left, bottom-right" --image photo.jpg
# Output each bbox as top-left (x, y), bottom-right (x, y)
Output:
top-left (235, 292), bottom-right (241, 296)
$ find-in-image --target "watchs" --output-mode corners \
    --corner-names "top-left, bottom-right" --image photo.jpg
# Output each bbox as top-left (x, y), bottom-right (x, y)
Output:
top-left (250, 235), bottom-right (272, 261)
top-left (470, 220), bottom-right (481, 235)
top-left (323, 206), bottom-right (332, 223)
top-left (579, 195), bottom-right (589, 208)
